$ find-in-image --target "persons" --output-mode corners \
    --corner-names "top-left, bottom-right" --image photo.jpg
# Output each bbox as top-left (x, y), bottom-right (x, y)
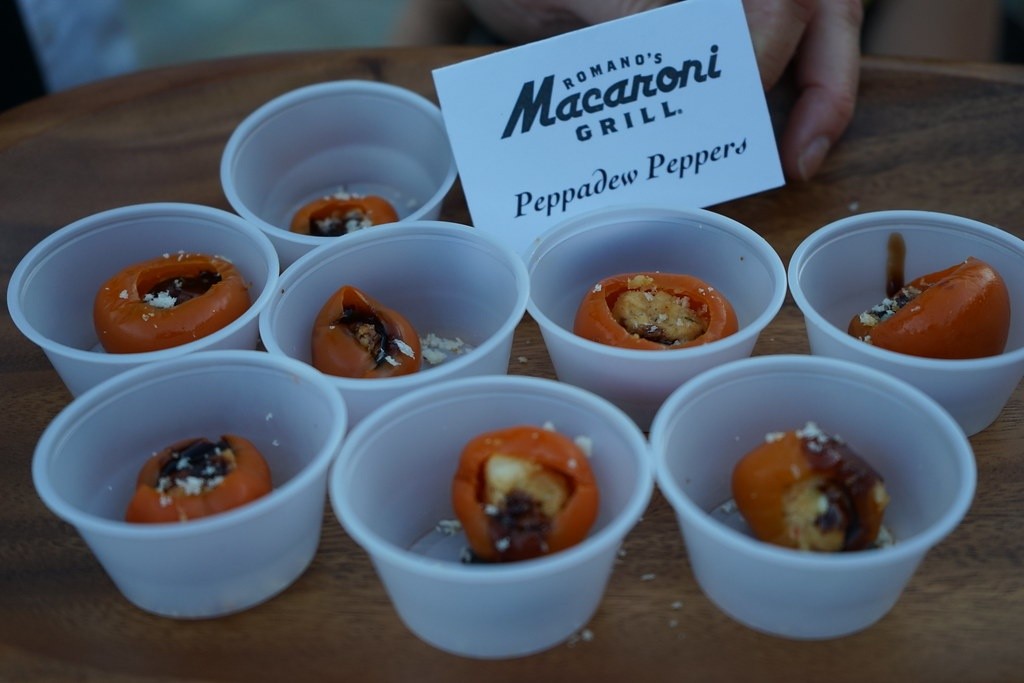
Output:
top-left (469, 0), bottom-right (865, 183)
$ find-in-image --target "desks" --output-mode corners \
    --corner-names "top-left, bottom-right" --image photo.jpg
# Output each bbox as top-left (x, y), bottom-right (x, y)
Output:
top-left (1, 48), bottom-right (1024, 683)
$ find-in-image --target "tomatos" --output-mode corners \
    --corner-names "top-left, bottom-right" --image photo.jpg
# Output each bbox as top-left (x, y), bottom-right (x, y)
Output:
top-left (92, 195), bottom-right (1010, 563)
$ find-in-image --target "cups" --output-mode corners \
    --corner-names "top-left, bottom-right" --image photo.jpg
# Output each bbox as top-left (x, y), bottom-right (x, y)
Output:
top-left (524, 200), bottom-right (786, 434)
top-left (218, 77), bottom-right (460, 271)
top-left (786, 208), bottom-right (1022, 440)
top-left (326, 374), bottom-right (656, 660)
top-left (32, 348), bottom-right (347, 619)
top-left (259, 220), bottom-right (531, 432)
top-left (651, 353), bottom-right (978, 639)
top-left (7, 202), bottom-right (280, 401)
